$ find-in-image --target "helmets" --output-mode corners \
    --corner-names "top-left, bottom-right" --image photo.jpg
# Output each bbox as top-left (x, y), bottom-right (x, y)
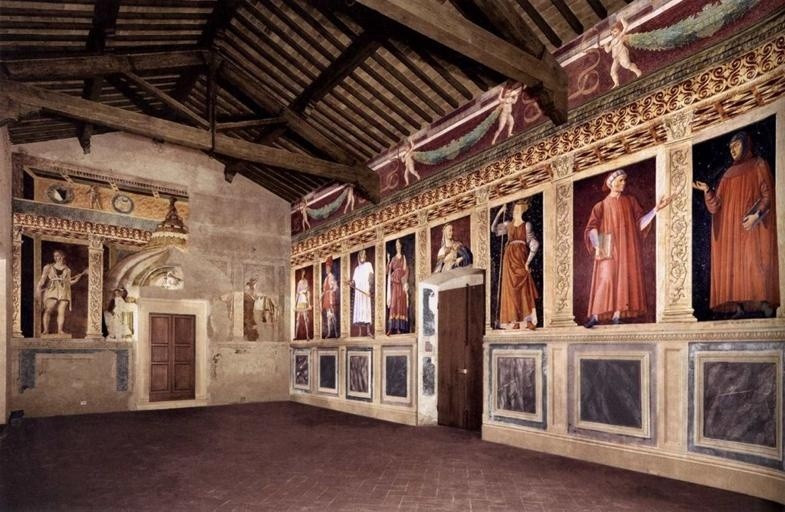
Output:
top-left (730, 132), bottom-right (748, 159)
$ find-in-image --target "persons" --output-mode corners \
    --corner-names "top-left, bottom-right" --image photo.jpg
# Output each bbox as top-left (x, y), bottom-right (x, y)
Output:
top-left (244, 277), bottom-right (256, 333)
top-left (433, 223), bottom-right (472, 274)
top-left (36, 249), bottom-right (81, 334)
top-left (110, 286), bottom-right (128, 321)
top-left (320, 256), bottom-right (339, 339)
top-left (342, 187), bottom-right (354, 215)
top-left (385, 238), bottom-right (409, 336)
top-left (692, 132), bottom-right (780, 318)
top-left (85, 184), bottom-right (102, 210)
top-left (584, 168), bottom-right (672, 328)
top-left (292, 269), bottom-right (312, 340)
top-left (490, 199), bottom-right (540, 330)
top-left (346, 250), bottom-right (374, 339)
top-left (491, 84), bottom-right (518, 146)
top-left (296, 198), bottom-right (311, 230)
top-left (400, 141), bottom-right (421, 188)
top-left (603, 17), bottom-right (642, 89)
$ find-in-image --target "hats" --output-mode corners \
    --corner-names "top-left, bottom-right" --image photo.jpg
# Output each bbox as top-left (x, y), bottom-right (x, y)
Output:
top-left (601, 170), bottom-right (625, 191)
